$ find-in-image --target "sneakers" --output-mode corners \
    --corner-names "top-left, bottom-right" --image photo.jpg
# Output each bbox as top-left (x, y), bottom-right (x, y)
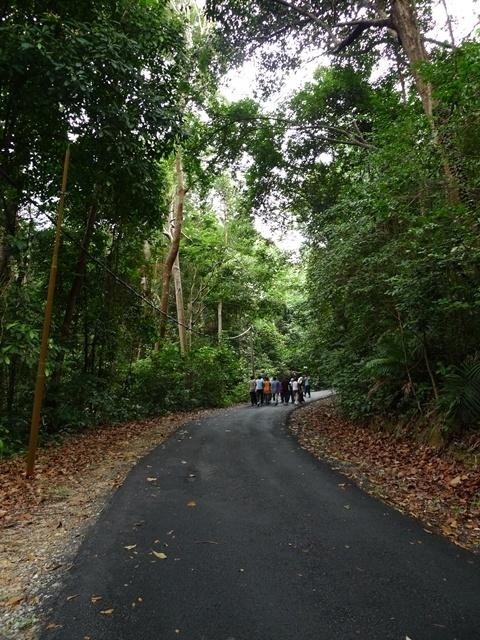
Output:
top-left (305, 395), bottom-right (311, 398)
top-left (252, 399), bottom-right (306, 407)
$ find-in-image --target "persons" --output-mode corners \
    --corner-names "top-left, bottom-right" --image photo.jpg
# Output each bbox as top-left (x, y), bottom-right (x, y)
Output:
top-left (248, 373), bottom-right (311, 406)
